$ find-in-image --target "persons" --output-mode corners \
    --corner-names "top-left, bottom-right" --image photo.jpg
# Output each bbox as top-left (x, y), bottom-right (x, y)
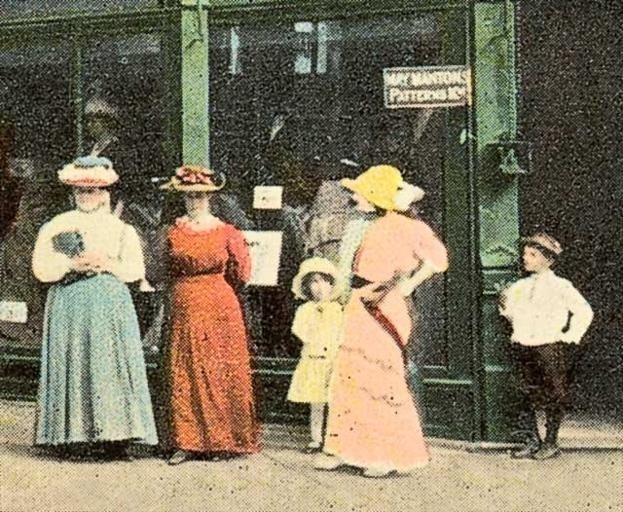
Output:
top-left (84, 96), bottom-right (139, 219)
top-left (146, 164), bottom-right (260, 466)
top-left (313, 164), bottom-right (449, 478)
top-left (496, 231), bottom-right (594, 460)
top-left (285, 256), bottom-right (344, 454)
top-left (30, 157), bottom-right (159, 462)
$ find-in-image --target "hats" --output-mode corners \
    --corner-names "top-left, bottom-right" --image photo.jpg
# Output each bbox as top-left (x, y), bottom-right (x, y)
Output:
top-left (175, 166), bottom-right (226, 192)
top-left (59, 155), bottom-right (121, 187)
top-left (341, 163), bottom-right (419, 212)
top-left (289, 257), bottom-right (342, 302)
top-left (521, 231), bottom-right (565, 255)
